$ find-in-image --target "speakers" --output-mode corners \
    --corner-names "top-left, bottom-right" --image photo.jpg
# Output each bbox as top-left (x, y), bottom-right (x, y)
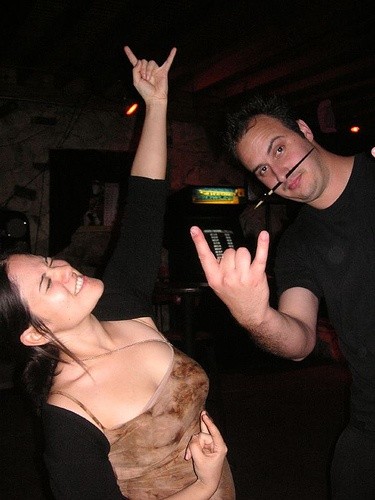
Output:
top-left (0, 210), bottom-right (31, 258)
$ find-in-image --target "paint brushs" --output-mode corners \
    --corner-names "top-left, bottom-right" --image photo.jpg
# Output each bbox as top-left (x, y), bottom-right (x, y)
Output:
top-left (251, 146), bottom-right (318, 213)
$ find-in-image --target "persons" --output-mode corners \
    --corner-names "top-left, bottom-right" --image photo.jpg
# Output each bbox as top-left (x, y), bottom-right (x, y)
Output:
top-left (189, 99), bottom-right (375, 500)
top-left (0, 46), bottom-right (236, 500)
